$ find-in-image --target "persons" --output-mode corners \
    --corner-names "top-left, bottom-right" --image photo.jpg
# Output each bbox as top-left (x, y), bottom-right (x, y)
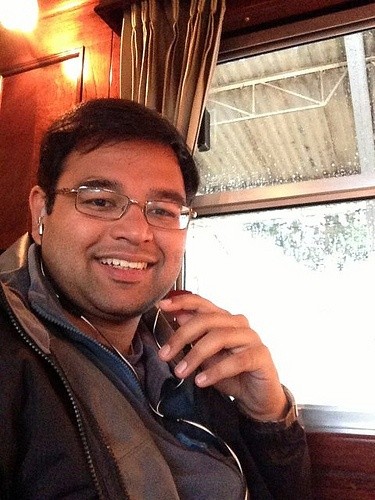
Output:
top-left (2, 99), bottom-right (315, 500)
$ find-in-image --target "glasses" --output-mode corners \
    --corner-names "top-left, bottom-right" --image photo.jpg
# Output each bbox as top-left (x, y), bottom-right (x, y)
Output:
top-left (48, 183), bottom-right (198, 231)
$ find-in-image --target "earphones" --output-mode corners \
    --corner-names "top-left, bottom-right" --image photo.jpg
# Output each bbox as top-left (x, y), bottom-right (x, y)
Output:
top-left (37, 208), bottom-right (44, 234)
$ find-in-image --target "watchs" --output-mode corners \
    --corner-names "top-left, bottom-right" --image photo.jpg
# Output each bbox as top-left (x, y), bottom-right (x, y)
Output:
top-left (233, 384), bottom-right (300, 434)
